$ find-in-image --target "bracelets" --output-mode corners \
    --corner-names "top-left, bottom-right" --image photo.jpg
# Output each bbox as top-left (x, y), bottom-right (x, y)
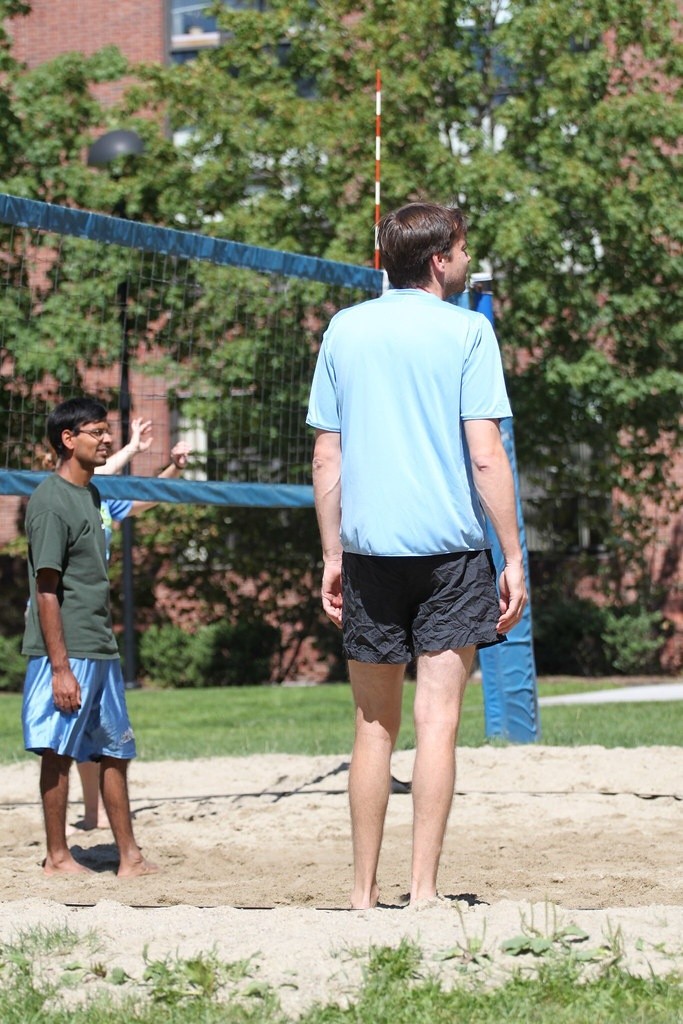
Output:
top-left (175, 463), bottom-right (185, 470)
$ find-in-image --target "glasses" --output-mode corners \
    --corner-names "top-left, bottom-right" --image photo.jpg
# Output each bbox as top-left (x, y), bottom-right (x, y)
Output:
top-left (73, 428), bottom-right (112, 437)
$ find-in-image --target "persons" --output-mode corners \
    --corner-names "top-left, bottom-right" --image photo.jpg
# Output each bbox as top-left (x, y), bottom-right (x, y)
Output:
top-left (53, 414), bottom-right (189, 828)
top-left (19, 397), bottom-right (166, 878)
top-left (305, 199), bottom-right (530, 908)
